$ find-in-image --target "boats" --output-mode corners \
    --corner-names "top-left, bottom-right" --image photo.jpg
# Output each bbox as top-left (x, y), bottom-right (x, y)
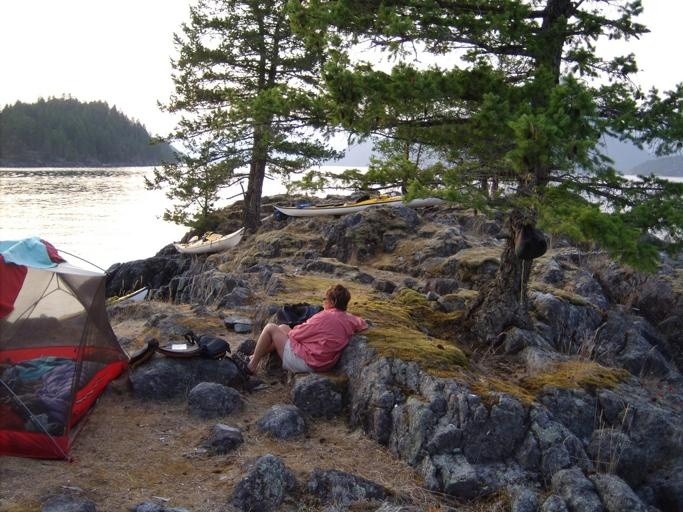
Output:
top-left (174, 227), bottom-right (246, 255)
top-left (268, 195), bottom-right (444, 217)
top-left (58, 286), bottom-right (151, 323)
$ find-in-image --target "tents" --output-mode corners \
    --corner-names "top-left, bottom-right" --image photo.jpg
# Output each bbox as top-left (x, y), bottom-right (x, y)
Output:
top-left (1, 236), bottom-right (129, 461)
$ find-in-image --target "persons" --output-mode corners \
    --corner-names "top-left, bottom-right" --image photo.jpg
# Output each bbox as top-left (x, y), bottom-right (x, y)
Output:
top-left (246, 284), bottom-right (369, 377)
